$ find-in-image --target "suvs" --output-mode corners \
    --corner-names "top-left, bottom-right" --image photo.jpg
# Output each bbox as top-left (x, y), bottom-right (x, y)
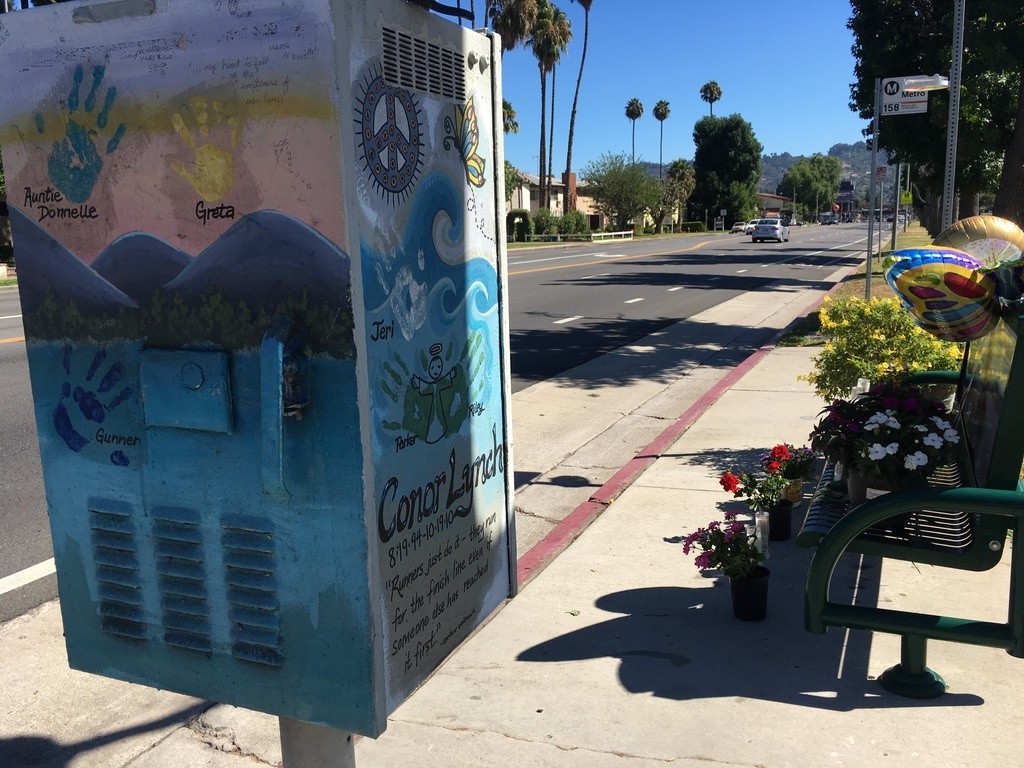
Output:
top-left (746, 219), bottom-right (761, 235)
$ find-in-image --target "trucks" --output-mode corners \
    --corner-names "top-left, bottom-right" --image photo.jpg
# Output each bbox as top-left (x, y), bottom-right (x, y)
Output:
top-left (820, 212), bottom-right (837, 224)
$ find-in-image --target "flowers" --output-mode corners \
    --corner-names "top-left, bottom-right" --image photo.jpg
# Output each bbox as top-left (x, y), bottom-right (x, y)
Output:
top-left (719, 443), bottom-right (818, 512)
top-left (683, 514), bottom-right (766, 571)
top-left (806, 380), bottom-right (960, 480)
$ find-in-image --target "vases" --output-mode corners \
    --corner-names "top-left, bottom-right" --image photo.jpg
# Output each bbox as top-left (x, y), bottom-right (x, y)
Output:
top-left (756, 498), bottom-right (791, 542)
top-left (846, 470), bottom-right (914, 534)
top-left (755, 511), bottom-right (769, 554)
top-left (783, 479), bottom-right (802, 508)
top-left (728, 565), bottom-right (770, 622)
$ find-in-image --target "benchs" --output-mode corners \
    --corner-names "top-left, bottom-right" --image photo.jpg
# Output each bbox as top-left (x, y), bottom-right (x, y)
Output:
top-left (797, 302), bottom-right (1024, 700)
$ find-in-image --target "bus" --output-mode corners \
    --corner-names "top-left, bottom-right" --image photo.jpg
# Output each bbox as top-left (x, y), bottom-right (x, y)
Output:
top-left (766, 212), bottom-right (780, 218)
top-left (898, 209), bottom-right (911, 221)
top-left (862, 208), bottom-right (894, 222)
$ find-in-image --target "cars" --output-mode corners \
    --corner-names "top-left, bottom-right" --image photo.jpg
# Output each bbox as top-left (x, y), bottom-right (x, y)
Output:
top-left (752, 218), bottom-right (790, 242)
top-left (732, 221), bottom-right (746, 233)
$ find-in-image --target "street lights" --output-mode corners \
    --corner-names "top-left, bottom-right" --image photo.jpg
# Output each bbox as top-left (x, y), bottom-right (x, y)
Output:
top-left (793, 185), bottom-right (803, 214)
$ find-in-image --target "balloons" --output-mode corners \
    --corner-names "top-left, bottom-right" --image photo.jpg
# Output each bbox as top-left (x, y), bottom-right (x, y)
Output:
top-left (881, 216), bottom-right (1024, 342)
